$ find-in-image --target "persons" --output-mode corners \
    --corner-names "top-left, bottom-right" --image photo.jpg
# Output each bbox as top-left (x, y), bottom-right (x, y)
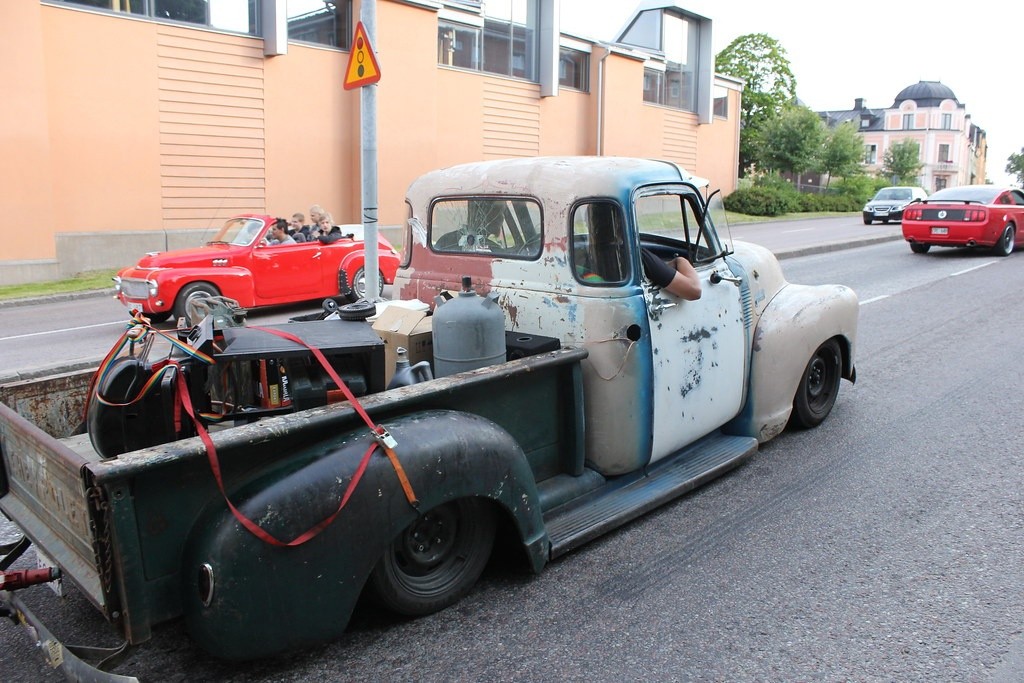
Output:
top-left (433, 198), bottom-right (507, 255)
top-left (286, 213), bottom-right (314, 243)
top-left (575, 201), bottom-right (703, 294)
top-left (267, 217), bottom-right (297, 246)
top-left (306, 204), bottom-right (325, 242)
top-left (311, 211), bottom-right (342, 242)
top-left (247, 222), bottom-right (270, 245)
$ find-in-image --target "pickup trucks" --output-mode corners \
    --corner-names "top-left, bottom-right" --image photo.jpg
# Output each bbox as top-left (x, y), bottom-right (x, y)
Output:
top-left (0, 157), bottom-right (857, 683)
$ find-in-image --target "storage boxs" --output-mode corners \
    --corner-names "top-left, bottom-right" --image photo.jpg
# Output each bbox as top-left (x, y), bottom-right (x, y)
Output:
top-left (371, 306), bottom-right (435, 390)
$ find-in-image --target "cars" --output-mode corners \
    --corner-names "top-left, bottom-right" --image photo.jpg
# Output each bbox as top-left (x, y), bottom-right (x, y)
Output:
top-left (110, 216), bottom-right (402, 326)
top-left (862, 186), bottom-right (927, 224)
top-left (901, 184), bottom-right (1024, 256)
